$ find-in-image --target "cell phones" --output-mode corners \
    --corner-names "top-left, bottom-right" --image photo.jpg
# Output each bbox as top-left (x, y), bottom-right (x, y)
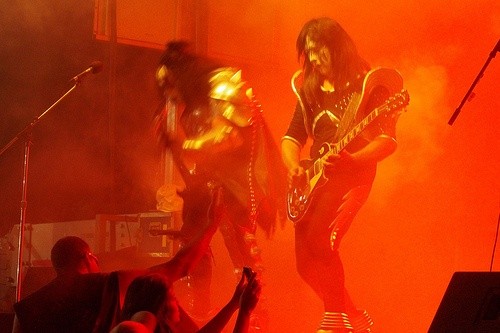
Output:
top-left (243, 266), bottom-right (257, 287)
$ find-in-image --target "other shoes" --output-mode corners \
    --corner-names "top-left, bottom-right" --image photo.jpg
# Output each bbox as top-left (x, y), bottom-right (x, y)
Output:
top-left (315, 309), bottom-right (378, 332)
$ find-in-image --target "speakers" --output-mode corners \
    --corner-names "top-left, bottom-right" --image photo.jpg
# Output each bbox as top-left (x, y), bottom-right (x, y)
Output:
top-left (428, 272), bottom-right (500, 333)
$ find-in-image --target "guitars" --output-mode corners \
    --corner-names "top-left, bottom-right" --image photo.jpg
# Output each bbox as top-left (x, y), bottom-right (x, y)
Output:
top-left (154, 112), bottom-right (203, 224)
top-left (287, 89), bottom-right (409, 224)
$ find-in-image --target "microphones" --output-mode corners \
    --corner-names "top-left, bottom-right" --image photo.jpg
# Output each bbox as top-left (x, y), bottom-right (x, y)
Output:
top-left (68, 61), bottom-right (103, 83)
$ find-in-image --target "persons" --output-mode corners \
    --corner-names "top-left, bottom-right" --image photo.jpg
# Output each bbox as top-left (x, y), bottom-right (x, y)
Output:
top-left (278, 16), bottom-right (411, 332)
top-left (0, 235), bottom-right (276, 333)
top-left (150, 38), bottom-right (286, 323)
